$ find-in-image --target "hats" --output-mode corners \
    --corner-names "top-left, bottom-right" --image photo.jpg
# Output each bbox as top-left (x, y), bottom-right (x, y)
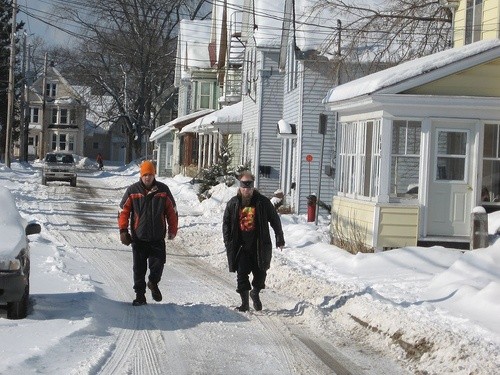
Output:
top-left (141, 161), bottom-right (156, 176)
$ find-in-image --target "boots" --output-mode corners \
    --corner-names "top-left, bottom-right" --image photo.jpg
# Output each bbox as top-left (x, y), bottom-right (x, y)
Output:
top-left (250, 286), bottom-right (262, 311)
top-left (236, 290), bottom-right (249, 312)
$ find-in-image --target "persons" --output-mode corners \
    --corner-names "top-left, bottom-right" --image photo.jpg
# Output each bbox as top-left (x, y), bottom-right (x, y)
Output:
top-left (118, 160), bottom-right (178, 306)
top-left (96, 153), bottom-right (103, 171)
top-left (222, 170), bottom-right (286, 312)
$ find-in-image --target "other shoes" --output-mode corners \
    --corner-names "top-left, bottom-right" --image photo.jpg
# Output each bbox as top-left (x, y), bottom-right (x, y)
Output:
top-left (148, 280), bottom-right (162, 301)
top-left (132, 293), bottom-right (146, 306)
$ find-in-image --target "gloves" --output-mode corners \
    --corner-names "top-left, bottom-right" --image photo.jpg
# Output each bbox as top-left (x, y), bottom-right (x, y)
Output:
top-left (120, 230), bottom-right (133, 246)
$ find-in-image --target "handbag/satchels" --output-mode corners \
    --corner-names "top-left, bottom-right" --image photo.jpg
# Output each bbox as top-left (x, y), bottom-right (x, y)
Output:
top-left (100, 161), bottom-right (103, 167)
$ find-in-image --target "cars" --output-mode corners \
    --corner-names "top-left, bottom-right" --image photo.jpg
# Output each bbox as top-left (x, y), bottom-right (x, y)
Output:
top-left (0, 186), bottom-right (41, 319)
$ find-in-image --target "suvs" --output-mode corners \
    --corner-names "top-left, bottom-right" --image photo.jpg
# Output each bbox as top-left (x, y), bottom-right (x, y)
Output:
top-left (41, 151), bottom-right (77, 187)
